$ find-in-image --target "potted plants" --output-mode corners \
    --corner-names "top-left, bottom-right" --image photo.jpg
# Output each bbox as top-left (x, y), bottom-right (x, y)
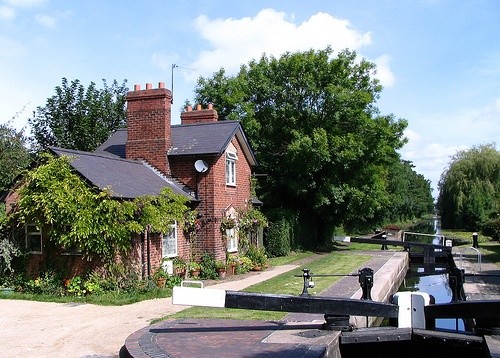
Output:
top-left (152, 247), bottom-right (270, 294)
